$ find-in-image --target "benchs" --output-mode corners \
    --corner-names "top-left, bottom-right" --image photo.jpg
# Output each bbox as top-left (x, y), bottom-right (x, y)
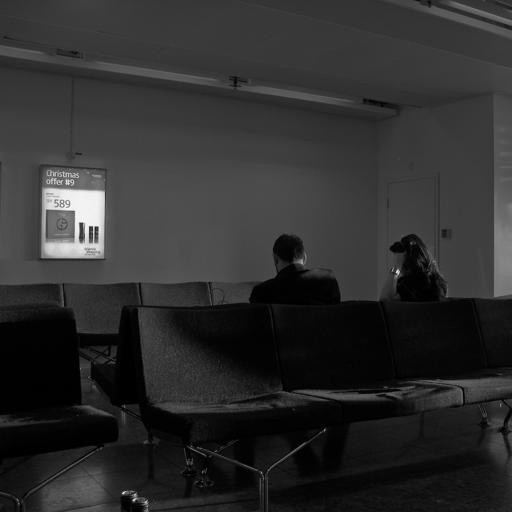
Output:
top-left (0, 279), bottom-right (512, 511)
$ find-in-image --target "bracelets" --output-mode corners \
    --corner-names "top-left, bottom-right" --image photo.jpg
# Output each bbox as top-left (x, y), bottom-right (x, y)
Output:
top-left (389, 267), bottom-right (400, 275)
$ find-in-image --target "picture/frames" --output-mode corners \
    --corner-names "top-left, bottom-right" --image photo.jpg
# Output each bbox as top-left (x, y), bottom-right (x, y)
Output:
top-left (39, 161), bottom-right (109, 263)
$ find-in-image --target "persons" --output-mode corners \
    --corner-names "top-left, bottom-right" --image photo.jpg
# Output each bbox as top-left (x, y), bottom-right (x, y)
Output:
top-left (380, 234), bottom-right (448, 301)
top-left (250, 233), bottom-right (340, 303)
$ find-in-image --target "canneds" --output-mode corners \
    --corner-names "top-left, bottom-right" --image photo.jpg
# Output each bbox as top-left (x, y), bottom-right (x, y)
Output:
top-left (121, 491), bottom-right (138, 512)
top-left (131, 497), bottom-right (148, 512)
